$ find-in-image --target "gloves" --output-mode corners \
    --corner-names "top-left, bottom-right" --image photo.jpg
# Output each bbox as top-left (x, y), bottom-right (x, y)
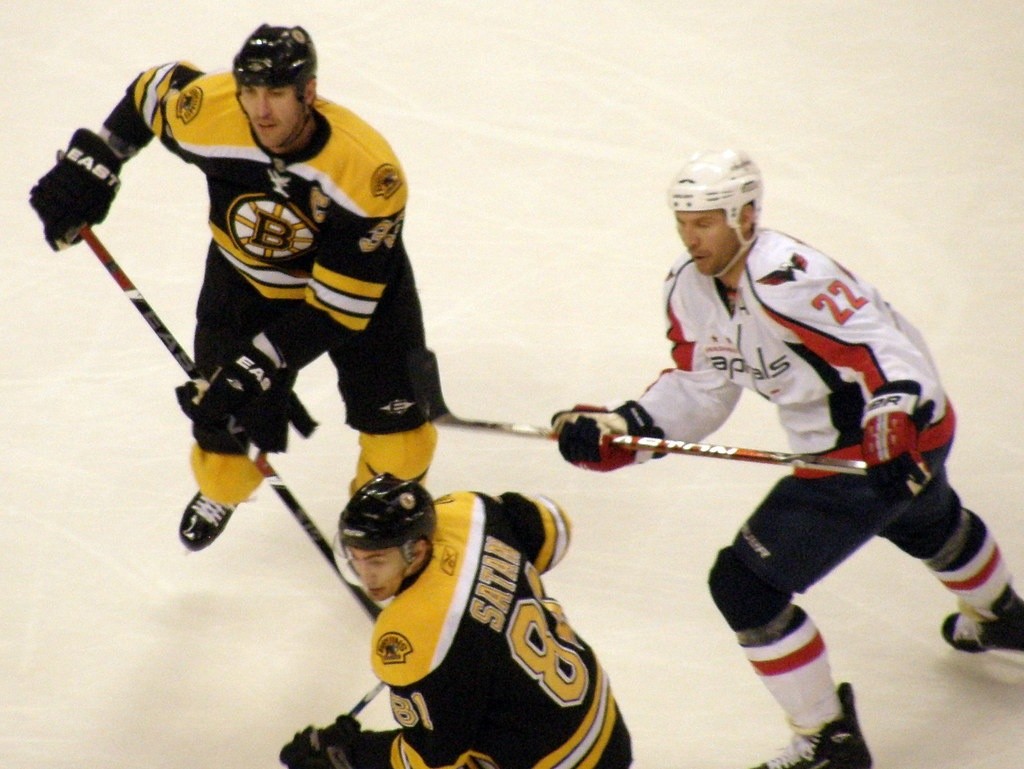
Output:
top-left (28, 127), bottom-right (125, 253)
top-left (278, 712), bottom-right (361, 769)
top-left (175, 328), bottom-right (287, 455)
top-left (861, 379), bottom-right (937, 508)
top-left (550, 400), bottom-right (668, 473)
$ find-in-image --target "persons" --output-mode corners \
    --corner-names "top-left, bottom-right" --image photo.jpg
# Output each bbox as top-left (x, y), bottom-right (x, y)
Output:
top-left (279, 471), bottom-right (633, 769)
top-left (28, 25), bottom-right (439, 552)
top-left (552, 147), bottom-right (1024, 769)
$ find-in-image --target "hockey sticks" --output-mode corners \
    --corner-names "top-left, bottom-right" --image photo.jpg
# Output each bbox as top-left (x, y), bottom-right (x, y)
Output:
top-left (408, 347), bottom-right (869, 478)
top-left (82, 223), bottom-right (389, 627)
top-left (347, 680), bottom-right (388, 720)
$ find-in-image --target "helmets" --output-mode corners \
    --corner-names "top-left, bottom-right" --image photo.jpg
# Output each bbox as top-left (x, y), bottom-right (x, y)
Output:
top-left (671, 145), bottom-right (766, 212)
top-left (336, 472), bottom-right (437, 549)
top-left (231, 23), bottom-right (318, 86)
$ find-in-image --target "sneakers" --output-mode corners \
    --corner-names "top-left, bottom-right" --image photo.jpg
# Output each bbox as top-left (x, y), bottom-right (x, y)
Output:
top-left (750, 682), bottom-right (873, 769)
top-left (939, 583), bottom-right (1024, 654)
top-left (177, 492), bottom-right (238, 554)
